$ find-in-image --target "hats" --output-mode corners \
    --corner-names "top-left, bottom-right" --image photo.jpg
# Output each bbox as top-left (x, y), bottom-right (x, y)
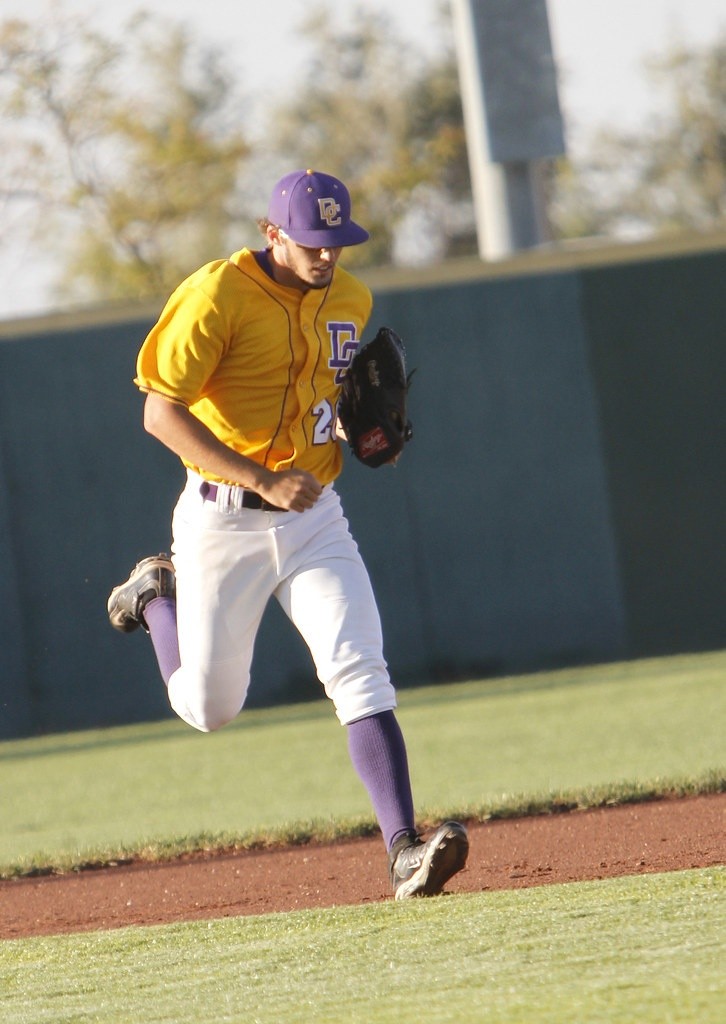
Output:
top-left (268, 168), bottom-right (369, 248)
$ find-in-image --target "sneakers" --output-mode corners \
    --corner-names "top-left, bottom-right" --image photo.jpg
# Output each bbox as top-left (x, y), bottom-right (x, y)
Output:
top-left (106, 553), bottom-right (176, 634)
top-left (387, 821), bottom-right (469, 901)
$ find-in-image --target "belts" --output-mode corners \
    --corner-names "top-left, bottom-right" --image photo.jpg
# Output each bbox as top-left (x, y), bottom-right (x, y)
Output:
top-left (200, 481), bottom-right (325, 512)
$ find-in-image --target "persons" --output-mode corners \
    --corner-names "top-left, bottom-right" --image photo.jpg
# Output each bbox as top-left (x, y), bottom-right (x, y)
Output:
top-left (108, 170), bottom-right (470, 900)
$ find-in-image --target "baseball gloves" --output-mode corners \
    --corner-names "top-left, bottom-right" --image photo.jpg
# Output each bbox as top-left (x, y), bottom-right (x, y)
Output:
top-left (337, 326), bottom-right (419, 468)
